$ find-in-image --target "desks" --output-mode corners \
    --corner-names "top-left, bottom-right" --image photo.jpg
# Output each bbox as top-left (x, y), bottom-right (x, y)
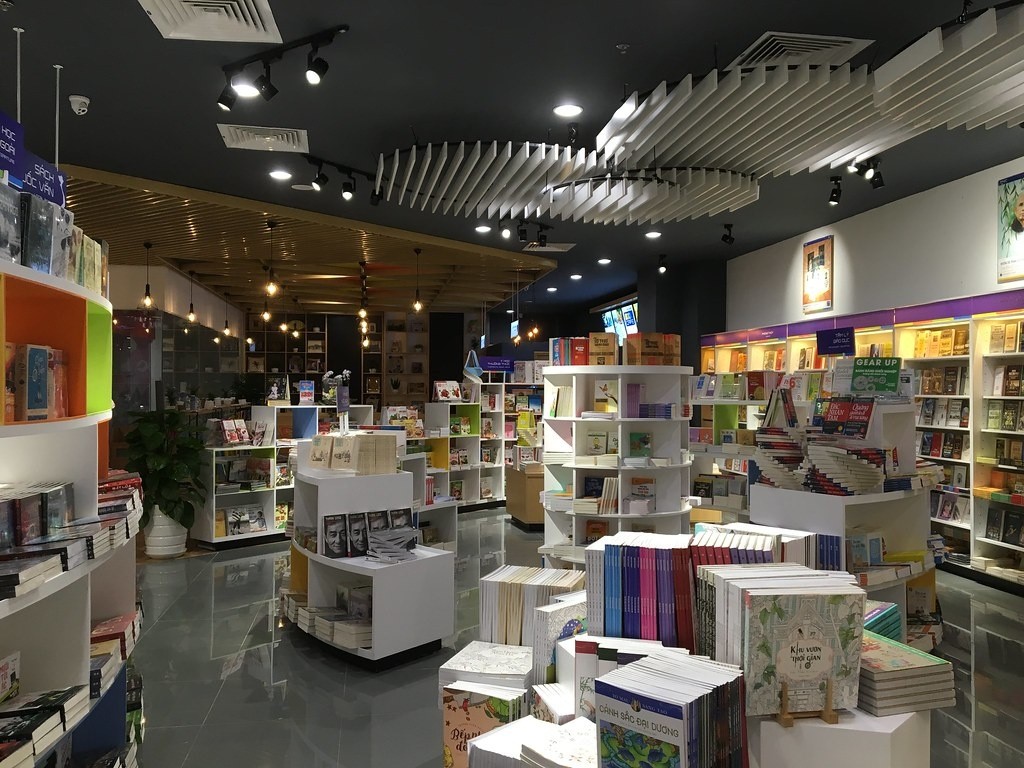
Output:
top-left (177, 403), bottom-right (252, 446)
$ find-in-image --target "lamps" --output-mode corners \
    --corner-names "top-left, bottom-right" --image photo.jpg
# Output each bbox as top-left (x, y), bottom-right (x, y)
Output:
top-left (871, 161), bottom-right (885, 189)
top-left (121, 408), bottom-right (207, 560)
top-left (218, 24), bottom-right (351, 116)
top-left (827, 176), bottom-right (843, 206)
top-left (497, 213), bottom-right (554, 247)
top-left (298, 152), bottom-right (384, 206)
top-left (720, 224), bottom-right (735, 244)
top-left (656, 254), bottom-right (667, 274)
top-left (855, 159), bottom-right (875, 180)
top-left (136, 220), bottom-right (422, 348)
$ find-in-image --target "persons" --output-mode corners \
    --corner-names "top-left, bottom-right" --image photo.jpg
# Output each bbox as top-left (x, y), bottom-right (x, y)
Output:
top-left (349, 518), bottom-right (369, 558)
top-left (325, 520), bottom-right (345, 558)
top-left (370, 515), bottom-right (389, 533)
top-left (393, 513), bottom-right (412, 528)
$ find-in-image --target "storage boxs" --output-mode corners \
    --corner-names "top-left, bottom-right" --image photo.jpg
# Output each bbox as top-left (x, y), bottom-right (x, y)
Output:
top-left (626, 332), bottom-right (681, 367)
top-left (513, 361), bottom-right (533, 383)
top-left (533, 359), bottom-right (550, 384)
top-left (588, 333), bottom-right (619, 365)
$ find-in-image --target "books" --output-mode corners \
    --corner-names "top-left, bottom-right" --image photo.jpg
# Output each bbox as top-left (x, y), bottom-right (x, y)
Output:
top-left (205, 418), bottom-right (274, 538)
top-left (266, 379), bottom-right (368, 528)
top-left (4, 341), bottom-right (68, 421)
top-left (370, 361), bottom-right (548, 550)
top-left (0, 182), bottom-right (108, 299)
top-left (538, 331), bottom-right (693, 571)
top-left (0, 593), bottom-right (145, 768)
top-left (0, 467), bottom-right (143, 598)
top-left (438, 520), bottom-right (956, 768)
top-left (278, 431), bottom-right (416, 649)
top-left (690, 318), bottom-right (1024, 585)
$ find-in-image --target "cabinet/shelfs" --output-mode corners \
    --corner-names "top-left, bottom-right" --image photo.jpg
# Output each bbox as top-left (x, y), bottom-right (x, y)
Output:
top-left (244, 313), bottom-right (328, 404)
top-left (688, 397), bottom-right (815, 536)
top-left (359, 310), bottom-right (430, 419)
top-left (456, 514), bottom-right (514, 638)
top-left (189, 363), bottom-right (694, 572)
top-left (748, 405), bottom-right (941, 654)
top-left (0, 255), bottom-right (160, 768)
top-left (930, 566), bottom-right (1024, 768)
top-left (282, 441), bottom-right (457, 673)
top-left (202, 548), bottom-right (291, 686)
top-left (698, 287), bottom-right (1024, 597)
